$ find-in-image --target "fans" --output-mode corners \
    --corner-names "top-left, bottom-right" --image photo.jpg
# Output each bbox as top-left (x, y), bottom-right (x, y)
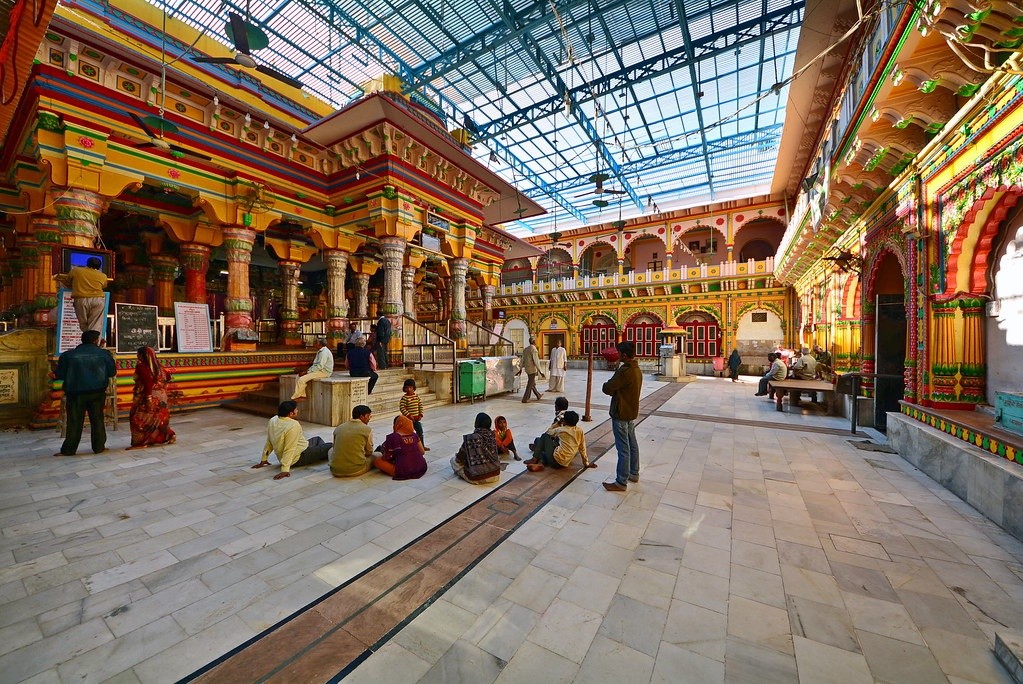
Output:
top-left (595, 121), bottom-right (637, 239)
top-left (127, 0), bottom-right (211, 161)
top-left (574, 0), bottom-right (626, 198)
top-left (189, 0), bottom-right (304, 89)
top-left (537, 113), bottom-right (572, 247)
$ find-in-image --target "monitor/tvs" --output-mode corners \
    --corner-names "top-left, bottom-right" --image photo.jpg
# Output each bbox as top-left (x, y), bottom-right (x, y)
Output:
top-left (62, 249), bottom-right (110, 277)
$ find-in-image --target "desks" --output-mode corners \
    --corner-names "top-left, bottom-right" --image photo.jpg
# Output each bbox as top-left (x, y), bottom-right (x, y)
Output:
top-left (768, 379), bottom-right (834, 411)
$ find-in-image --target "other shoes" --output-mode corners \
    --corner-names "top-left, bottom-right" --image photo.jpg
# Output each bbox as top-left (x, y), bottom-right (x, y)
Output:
top-left (94, 448), bottom-right (109, 453)
top-left (54, 453), bottom-right (65, 456)
top-left (125, 443), bottom-right (147, 450)
top-left (168, 435), bottom-right (176, 444)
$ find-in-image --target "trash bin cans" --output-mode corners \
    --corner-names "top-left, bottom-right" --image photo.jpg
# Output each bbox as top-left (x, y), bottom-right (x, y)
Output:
top-left (459, 361), bottom-right (485, 405)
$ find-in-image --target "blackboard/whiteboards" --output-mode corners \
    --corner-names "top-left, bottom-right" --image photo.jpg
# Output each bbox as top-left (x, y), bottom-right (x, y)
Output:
top-left (114, 302), bottom-right (160, 353)
top-left (55, 288), bottom-right (110, 357)
top-left (174, 302), bottom-right (214, 354)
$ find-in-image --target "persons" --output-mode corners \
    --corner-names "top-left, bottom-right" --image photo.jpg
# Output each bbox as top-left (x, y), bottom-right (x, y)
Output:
top-left (368, 324), bottom-right (378, 352)
top-left (754, 353), bottom-right (787, 396)
top-left (728, 349), bottom-right (741, 382)
top-left (494, 415), bottom-right (522, 461)
top-left (374, 415), bottom-right (427, 481)
top-left (53, 330), bottom-right (117, 457)
top-left (545, 339), bottom-right (567, 392)
top-left (376, 311), bottom-right (391, 370)
top-left (602, 341), bottom-right (643, 492)
top-left (328, 405), bottom-right (382, 478)
top-left (345, 336), bottom-right (378, 395)
top-left (793, 347), bottom-right (817, 380)
top-left (813, 345), bottom-right (835, 380)
top-left (125, 346), bottom-right (176, 450)
top-left (291, 339), bottom-right (334, 400)
top-left (64, 257), bottom-right (107, 349)
top-left (399, 379), bottom-right (430, 452)
top-left (514, 337), bottom-right (545, 403)
top-left (450, 412), bottom-right (500, 485)
top-left (251, 401), bottom-right (333, 480)
top-left (523, 396), bottom-right (599, 472)
top-left (335, 323), bottom-right (363, 362)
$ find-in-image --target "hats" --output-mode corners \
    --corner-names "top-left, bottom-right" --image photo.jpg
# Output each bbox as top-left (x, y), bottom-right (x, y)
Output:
top-left (802, 348), bottom-right (810, 353)
